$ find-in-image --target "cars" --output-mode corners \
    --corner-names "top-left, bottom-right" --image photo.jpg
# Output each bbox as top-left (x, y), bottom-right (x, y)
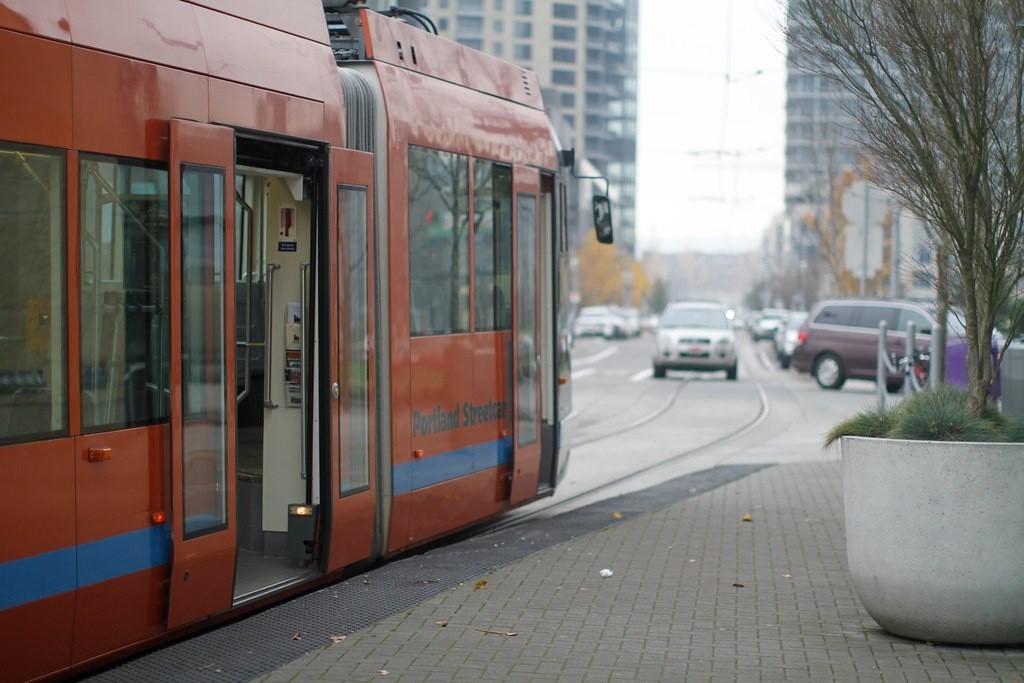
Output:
top-left (572, 305), bottom-right (641, 341)
top-left (751, 308), bottom-right (808, 370)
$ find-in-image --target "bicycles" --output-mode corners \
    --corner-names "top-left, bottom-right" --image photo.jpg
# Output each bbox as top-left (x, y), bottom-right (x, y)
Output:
top-left (890, 345), bottom-right (931, 385)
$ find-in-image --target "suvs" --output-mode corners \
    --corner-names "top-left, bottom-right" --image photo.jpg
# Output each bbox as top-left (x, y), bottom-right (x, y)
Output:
top-left (650, 298), bottom-right (741, 381)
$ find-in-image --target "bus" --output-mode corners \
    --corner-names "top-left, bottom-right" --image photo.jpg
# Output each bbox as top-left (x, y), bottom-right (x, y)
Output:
top-left (1, 0), bottom-right (618, 683)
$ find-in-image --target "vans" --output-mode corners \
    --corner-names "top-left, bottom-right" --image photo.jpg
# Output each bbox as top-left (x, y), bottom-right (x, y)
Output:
top-left (790, 297), bottom-right (1005, 391)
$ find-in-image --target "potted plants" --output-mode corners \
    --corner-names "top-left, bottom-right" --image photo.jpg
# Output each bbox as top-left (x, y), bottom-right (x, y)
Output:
top-left (776, 0), bottom-right (1024, 649)
